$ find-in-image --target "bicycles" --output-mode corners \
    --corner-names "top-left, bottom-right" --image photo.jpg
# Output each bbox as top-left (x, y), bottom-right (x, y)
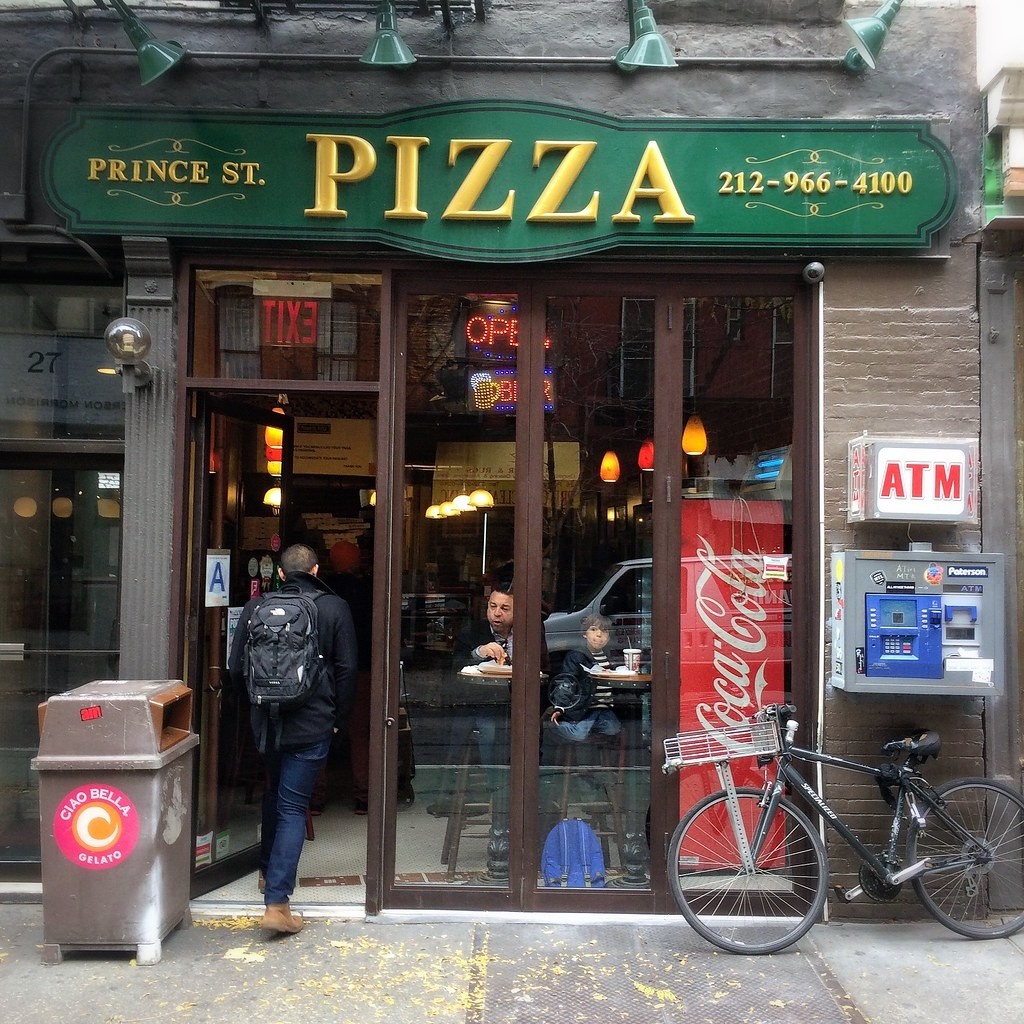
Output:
top-left (660, 702), bottom-right (1024, 956)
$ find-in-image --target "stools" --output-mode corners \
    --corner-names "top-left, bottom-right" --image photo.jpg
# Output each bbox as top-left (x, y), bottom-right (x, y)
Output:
top-left (441, 728), bottom-right (496, 875)
top-left (547, 734), bottom-right (626, 869)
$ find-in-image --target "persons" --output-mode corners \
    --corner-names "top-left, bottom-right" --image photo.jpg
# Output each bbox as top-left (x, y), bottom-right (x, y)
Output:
top-left (541, 613), bottom-right (621, 741)
top-left (310, 541), bottom-right (372, 815)
top-left (494, 557), bottom-right (514, 582)
top-left (449, 584), bottom-right (551, 798)
top-left (226, 544), bottom-right (357, 933)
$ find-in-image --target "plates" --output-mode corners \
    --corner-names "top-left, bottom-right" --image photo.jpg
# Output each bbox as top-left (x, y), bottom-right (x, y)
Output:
top-left (590, 672), bottom-right (636, 677)
top-left (478, 669), bottom-right (512, 674)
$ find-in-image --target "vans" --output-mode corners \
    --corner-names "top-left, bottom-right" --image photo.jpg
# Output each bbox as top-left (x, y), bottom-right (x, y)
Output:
top-left (543, 553), bottom-right (792, 711)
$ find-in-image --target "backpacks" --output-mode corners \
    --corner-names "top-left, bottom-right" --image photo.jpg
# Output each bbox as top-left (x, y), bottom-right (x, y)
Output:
top-left (542, 819), bottom-right (605, 888)
top-left (243, 584), bottom-right (328, 706)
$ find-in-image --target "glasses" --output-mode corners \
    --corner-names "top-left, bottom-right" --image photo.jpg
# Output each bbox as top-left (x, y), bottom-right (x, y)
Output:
top-left (501, 639), bottom-right (511, 665)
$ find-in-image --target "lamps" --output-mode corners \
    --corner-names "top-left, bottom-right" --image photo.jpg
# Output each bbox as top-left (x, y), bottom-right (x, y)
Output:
top-left (682, 397), bottom-right (712, 457)
top-left (64, 0), bottom-right (906, 88)
top-left (262, 487), bottom-right (282, 506)
top-left (600, 444), bottom-right (623, 484)
top-left (635, 434), bottom-right (655, 473)
top-left (264, 407), bottom-right (285, 449)
top-left (451, 494), bottom-right (478, 512)
top-left (267, 461), bottom-right (282, 476)
top-left (468, 483), bottom-right (499, 508)
top-left (369, 490), bottom-right (377, 506)
top-left (13, 497), bottom-right (120, 518)
top-left (424, 504), bottom-right (446, 521)
top-left (437, 500), bottom-right (462, 517)
top-left (265, 444), bottom-right (283, 462)
top-left (103, 315), bottom-right (154, 396)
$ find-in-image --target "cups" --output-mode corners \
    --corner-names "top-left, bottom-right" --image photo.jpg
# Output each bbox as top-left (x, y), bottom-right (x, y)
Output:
top-left (623, 649), bottom-right (642, 672)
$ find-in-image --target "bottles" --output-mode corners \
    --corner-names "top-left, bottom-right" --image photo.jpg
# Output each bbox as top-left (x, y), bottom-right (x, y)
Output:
top-left (488, 535), bottom-right (514, 575)
top-left (490, 518), bottom-right (514, 530)
top-left (459, 552), bottom-right (482, 585)
top-left (439, 535), bottom-right (476, 545)
top-left (437, 550), bottom-right (466, 563)
top-left (425, 560), bottom-right (439, 593)
top-left (442, 526), bottom-right (478, 534)
top-left (488, 507), bottom-right (514, 519)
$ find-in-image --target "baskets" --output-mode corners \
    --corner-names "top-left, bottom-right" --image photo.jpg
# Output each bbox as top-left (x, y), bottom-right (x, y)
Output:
top-left (663, 720), bottom-right (781, 775)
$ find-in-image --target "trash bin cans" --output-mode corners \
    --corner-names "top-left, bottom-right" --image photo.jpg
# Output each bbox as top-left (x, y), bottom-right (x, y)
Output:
top-left (29, 679), bottom-right (199, 966)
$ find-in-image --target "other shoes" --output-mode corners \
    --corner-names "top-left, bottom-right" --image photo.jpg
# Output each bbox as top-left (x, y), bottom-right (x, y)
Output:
top-left (306, 792), bottom-right (326, 816)
top-left (257, 871), bottom-right (268, 893)
top-left (539, 705), bottom-right (556, 730)
top-left (426, 793), bottom-right (457, 817)
top-left (262, 902), bottom-right (304, 934)
top-left (354, 787), bottom-right (369, 815)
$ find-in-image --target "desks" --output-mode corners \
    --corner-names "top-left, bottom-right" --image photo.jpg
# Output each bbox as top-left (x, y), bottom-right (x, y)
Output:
top-left (583, 673), bottom-right (653, 888)
top-left (454, 665), bottom-right (549, 886)
